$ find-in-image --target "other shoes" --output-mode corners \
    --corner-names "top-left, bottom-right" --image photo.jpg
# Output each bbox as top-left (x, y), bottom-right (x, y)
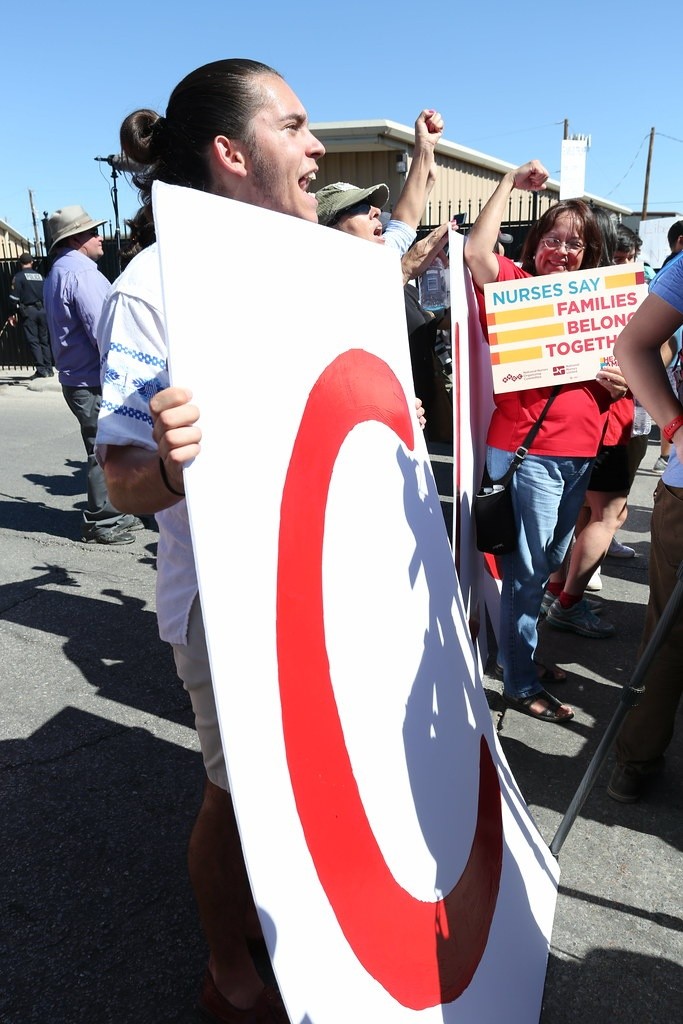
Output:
top-left (655, 458), bottom-right (669, 470)
top-left (31, 370), bottom-right (46, 380)
top-left (608, 757), bottom-right (668, 804)
top-left (82, 521), bottom-right (135, 545)
top-left (607, 538), bottom-right (636, 559)
top-left (126, 518), bottom-right (144, 531)
top-left (47, 372), bottom-right (54, 377)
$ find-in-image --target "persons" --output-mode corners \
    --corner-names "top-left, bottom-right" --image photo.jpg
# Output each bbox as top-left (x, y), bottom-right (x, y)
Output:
top-left (405, 158), bottom-right (682, 721)
top-left (315, 109), bottom-right (443, 254)
top-left (94, 59), bottom-right (426, 1024)
top-left (608, 253), bottom-right (683, 799)
top-left (9, 253), bottom-right (53, 379)
top-left (43, 206), bottom-right (144, 545)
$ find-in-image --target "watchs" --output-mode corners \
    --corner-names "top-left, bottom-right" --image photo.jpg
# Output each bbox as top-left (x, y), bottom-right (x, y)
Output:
top-left (662, 414), bottom-right (683, 444)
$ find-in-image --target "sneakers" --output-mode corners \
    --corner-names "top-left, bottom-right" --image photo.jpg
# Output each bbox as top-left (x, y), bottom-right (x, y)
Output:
top-left (547, 599), bottom-right (616, 639)
top-left (540, 590), bottom-right (603, 615)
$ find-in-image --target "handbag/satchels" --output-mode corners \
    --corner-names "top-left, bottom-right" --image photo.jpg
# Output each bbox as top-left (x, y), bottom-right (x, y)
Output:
top-left (475, 479), bottom-right (520, 554)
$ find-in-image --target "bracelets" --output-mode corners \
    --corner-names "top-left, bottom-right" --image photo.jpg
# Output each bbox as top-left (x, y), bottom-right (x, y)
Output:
top-left (160, 457), bottom-right (185, 497)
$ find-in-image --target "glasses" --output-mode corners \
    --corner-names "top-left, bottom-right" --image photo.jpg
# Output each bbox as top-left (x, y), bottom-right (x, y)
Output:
top-left (345, 199), bottom-right (371, 215)
top-left (89, 228), bottom-right (99, 237)
top-left (542, 237), bottom-right (586, 254)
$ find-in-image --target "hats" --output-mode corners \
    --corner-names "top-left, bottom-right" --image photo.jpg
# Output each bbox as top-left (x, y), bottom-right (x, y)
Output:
top-left (315, 182), bottom-right (389, 226)
top-left (48, 205), bottom-right (108, 255)
top-left (20, 253), bottom-right (34, 264)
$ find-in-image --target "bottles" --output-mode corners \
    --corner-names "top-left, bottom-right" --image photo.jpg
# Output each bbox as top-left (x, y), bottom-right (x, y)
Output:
top-left (632, 398), bottom-right (652, 435)
top-left (491, 485), bottom-right (503, 494)
top-left (417, 256), bottom-right (447, 311)
top-left (484, 488), bottom-right (492, 496)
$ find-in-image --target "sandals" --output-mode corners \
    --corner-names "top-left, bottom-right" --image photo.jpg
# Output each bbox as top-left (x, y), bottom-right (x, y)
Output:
top-left (502, 690), bottom-right (573, 722)
top-left (496, 663), bottom-right (564, 686)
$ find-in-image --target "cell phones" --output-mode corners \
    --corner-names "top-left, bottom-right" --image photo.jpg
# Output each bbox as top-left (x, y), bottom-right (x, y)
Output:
top-left (453, 213), bottom-right (467, 225)
